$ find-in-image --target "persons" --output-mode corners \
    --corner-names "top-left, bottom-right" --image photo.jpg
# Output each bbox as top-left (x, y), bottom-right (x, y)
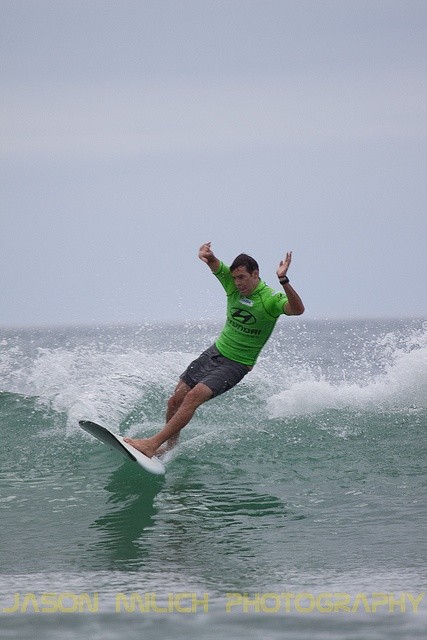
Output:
top-left (123, 241), bottom-right (305, 459)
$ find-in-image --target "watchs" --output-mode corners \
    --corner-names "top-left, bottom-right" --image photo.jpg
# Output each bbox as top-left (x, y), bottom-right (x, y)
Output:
top-left (279, 277), bottom-right (290, 285)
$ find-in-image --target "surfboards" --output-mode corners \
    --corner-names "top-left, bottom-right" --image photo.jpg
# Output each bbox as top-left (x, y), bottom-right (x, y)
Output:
top-left (76, 416), bottom-right (167, 477)
